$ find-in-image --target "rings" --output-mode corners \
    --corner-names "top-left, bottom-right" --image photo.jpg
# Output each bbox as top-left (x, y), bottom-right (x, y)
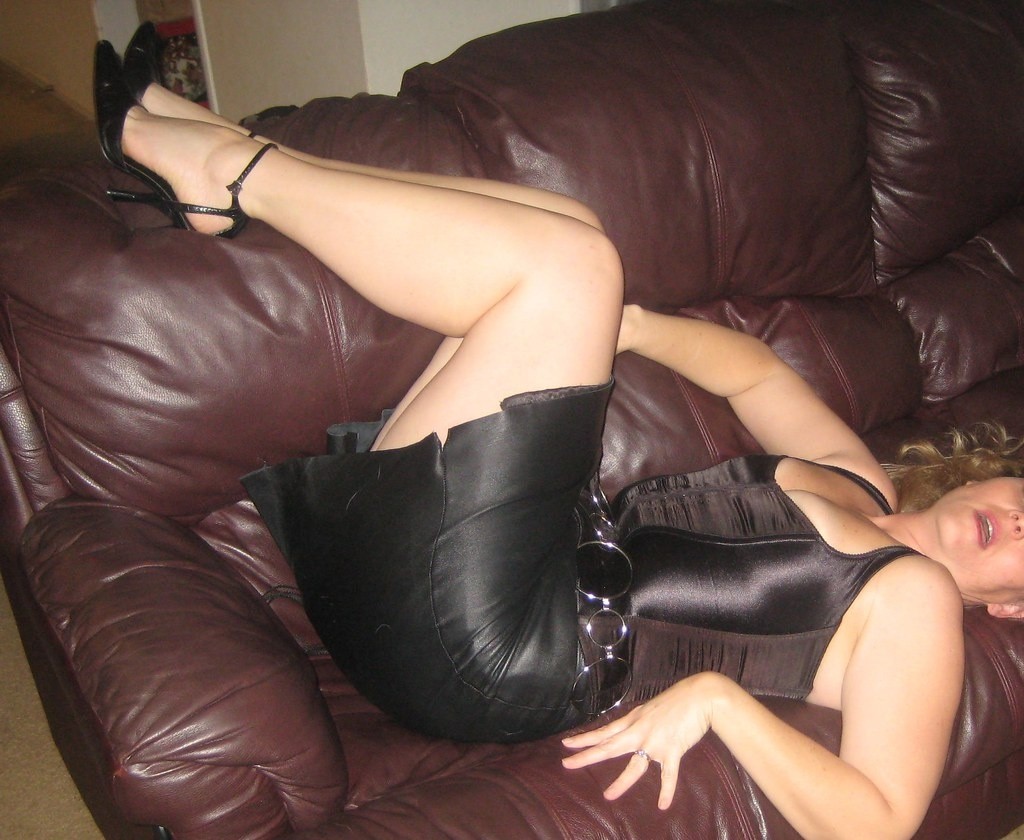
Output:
top-left (635, 750), bottom-right (652, 764)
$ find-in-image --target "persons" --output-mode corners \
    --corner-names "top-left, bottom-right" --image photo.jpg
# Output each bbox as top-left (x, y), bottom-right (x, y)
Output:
top-left (90, 22), bottom-right (1024, 839)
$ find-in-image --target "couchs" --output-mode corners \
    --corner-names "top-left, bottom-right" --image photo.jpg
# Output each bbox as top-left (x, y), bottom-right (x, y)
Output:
top-left (0, 0), bottom-right (1024, 840)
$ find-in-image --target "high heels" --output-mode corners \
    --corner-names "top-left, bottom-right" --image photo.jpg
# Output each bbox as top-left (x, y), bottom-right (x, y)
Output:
top-left (94, 41), bottom-right (277, 238)
top-left (123, 22), bottom-right (255, 138)
